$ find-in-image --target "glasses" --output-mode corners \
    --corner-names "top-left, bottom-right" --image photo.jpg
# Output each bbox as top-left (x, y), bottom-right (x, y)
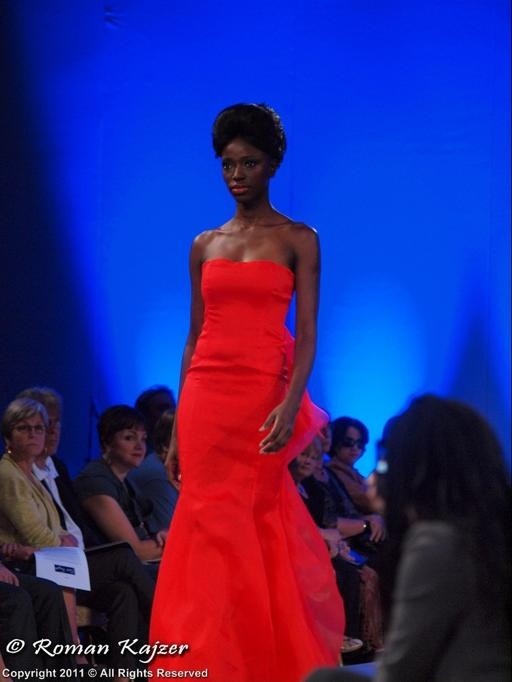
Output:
top-left (344, 438), bottom-right (363, 449)
top-left (16, 424), bottom-right (45, 434)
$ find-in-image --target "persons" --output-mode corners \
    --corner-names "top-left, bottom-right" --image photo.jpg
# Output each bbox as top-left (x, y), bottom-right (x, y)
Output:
top-left (145, 100), bottom-right (347, 682)
